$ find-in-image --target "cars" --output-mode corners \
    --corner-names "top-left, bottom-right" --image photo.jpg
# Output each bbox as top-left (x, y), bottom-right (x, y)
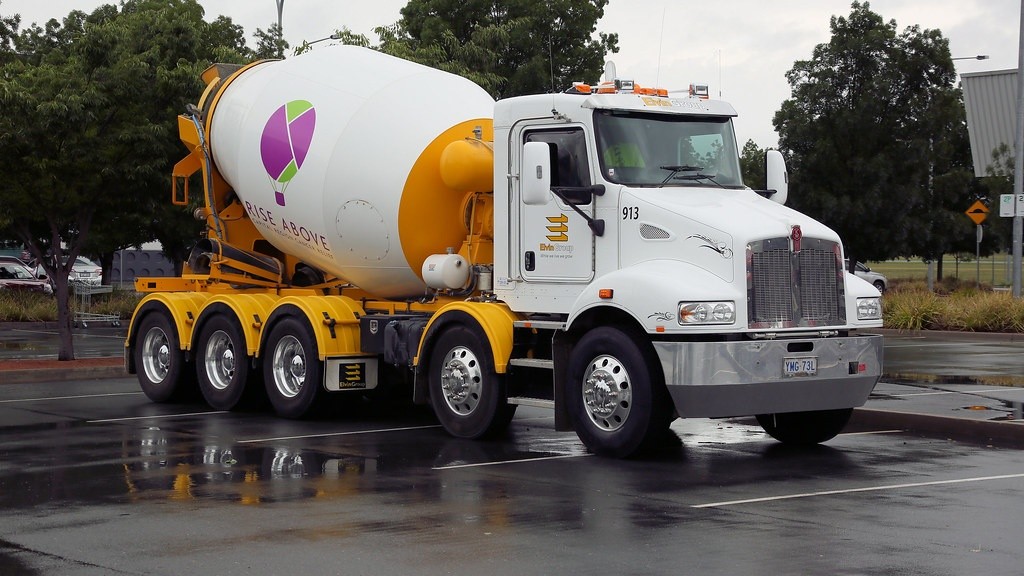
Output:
top-left (845, 259), bottom-right (888, 295)
top-left (28, 256), bottom-right (103, 286)
top-left (0, 256), bottom-right (34, 275)
top-left (0, 263), bottom-right (52, 295)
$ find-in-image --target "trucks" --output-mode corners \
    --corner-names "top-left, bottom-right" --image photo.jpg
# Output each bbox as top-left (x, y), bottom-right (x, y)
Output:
top-left (126, 45), bottom-right (884, 456)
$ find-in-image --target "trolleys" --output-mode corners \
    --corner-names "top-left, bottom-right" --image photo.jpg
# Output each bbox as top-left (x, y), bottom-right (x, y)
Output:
top-left (72, 278), bottom-right (121, 329)
